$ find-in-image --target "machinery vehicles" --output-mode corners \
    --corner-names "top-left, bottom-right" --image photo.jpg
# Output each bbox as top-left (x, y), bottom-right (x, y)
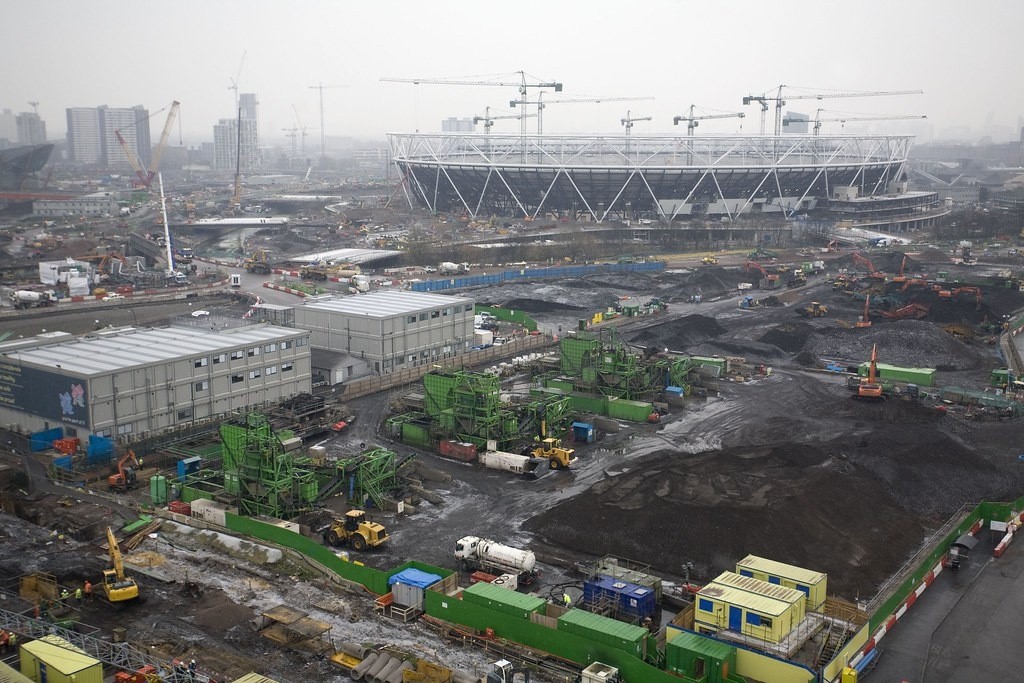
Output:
top-left (528, 408), bottom-right (579, 470)
top-left (852, 340), bottom-right (891, 402)
top-left (793, 300), bottom-right (830, 318)
top-left (321, 508), bottom-right (391, 555)
top-left (701, 251), bottom-right (983, 312)
top-left (855, 294), bottom-right (873, 329)
top-left (101, 524), bottom-right (139, 602)
top-left (106, 449), bottom-right (141, 492)
top-left (453, 534), bottom-right (538, 584)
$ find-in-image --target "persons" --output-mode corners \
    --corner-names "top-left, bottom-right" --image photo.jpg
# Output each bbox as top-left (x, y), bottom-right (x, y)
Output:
top-left (562, 593), bottom-right (571, 607)
top-left (367, 234), bottom-right (369, 240)
top-left (215, 256), bottom-right (244, 267)
top-left (1003, 382), bottom-right (1007, 394)
top-left (260, 219), bottom-right (265, 223)
top-left (8, 575), bottom-right (197, 677)
top-left (513, 325), bottom-right (561, 339)
top-left (813, 304), bottom-right (819, 311)
top-left (139, 457), bottom-right (144, 471)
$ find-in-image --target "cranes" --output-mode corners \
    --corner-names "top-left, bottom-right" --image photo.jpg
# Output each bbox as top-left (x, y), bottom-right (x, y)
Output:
top-left (112, 99), bottom-right (183, 188)
top-left (278, 81), bottom-right (353, 168)
top-left (226, 46), bottom-right (250, 99)
top-left (376, 68), bottom-right (933, 160)
